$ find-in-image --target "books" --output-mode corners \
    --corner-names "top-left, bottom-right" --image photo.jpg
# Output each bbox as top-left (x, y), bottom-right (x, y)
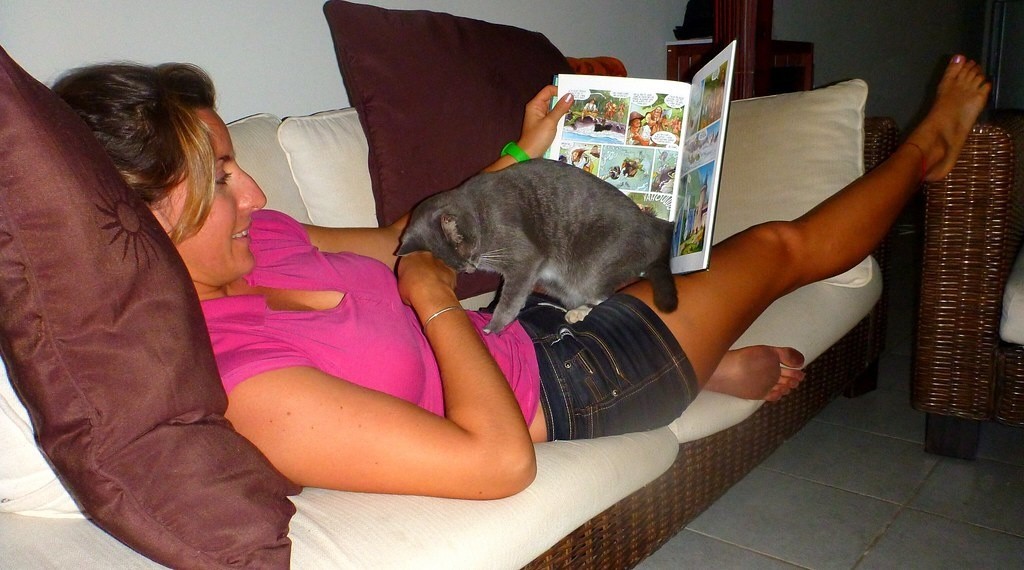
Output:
top-left (539, 38), bottom-right (738, 272)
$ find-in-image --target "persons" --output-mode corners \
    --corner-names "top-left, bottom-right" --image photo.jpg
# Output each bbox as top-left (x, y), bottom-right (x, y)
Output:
top-left (51, 53), bottom-right (992, 499)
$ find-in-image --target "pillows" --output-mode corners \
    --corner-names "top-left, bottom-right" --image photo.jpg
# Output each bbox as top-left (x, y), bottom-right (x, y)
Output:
top-left (323, 0), bottom-right (574, 301)
top-left (278, 105), bottom-right (378, 230)
top-left (223, 113), bottom-right (313, 227)
top-left (713, 78), bottom-right (873, 287)
top-left (0, 46), bottom-right (303, 570)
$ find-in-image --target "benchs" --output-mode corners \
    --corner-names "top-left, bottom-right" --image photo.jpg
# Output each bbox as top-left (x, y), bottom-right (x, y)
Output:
top-left (0, 81), bottom-right (1023, 570)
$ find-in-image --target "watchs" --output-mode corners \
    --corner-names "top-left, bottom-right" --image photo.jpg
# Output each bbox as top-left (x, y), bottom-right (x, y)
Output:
top-left (499, 141), bottom-right (532, 163)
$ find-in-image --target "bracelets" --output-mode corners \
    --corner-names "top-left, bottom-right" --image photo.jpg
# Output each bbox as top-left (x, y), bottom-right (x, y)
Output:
top-left (422, 304), bottom-right (463, 335)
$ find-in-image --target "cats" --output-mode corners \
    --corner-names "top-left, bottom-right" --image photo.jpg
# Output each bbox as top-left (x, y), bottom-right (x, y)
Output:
top-left (396, 157), bottom-right (678, 334)
